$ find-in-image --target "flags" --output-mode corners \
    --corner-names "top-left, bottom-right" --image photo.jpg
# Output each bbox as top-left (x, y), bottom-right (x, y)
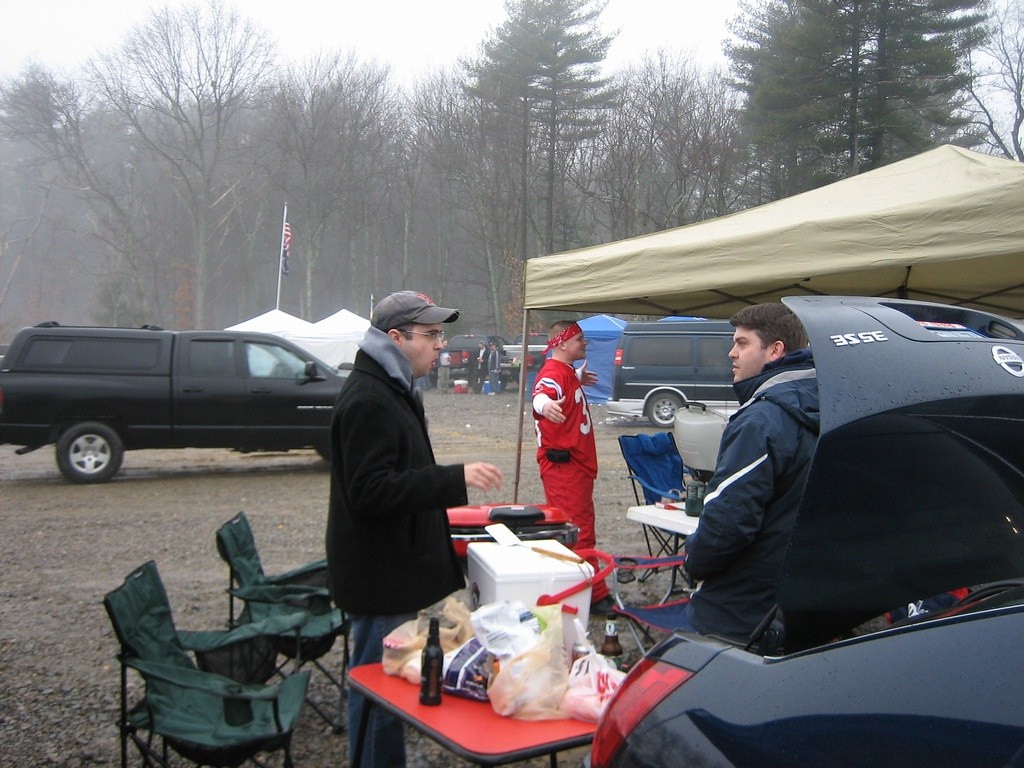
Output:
top-left (282, 215), bottom-right (292, 275)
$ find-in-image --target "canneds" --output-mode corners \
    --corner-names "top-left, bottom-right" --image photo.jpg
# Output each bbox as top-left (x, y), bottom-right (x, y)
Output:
top-left (685, 481), bottom-right (704, 517)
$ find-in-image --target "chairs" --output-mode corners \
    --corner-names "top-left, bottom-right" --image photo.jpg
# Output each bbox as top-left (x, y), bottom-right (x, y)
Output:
top-left (618, 432), bottom-right (705, 589)
top-left (215, 511), bottom-right (351, 734)
top-left (103, 561), bottom-right (311, 768)
top-left (604, 552), bottom-right (698, 656)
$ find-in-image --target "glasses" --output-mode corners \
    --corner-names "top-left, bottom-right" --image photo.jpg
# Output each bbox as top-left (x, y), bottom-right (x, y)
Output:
top-left (397, 329), bottom-right (444, 341)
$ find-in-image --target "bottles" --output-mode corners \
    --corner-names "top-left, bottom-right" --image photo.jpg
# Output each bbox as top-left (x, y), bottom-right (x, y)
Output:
top-left (419, 618), bottom-right (444, 705)
top-left (559, 603), bottom-right (579, 670)
top-left (685, 477), bottom-right (706, 516)
top-left (602, 635), bottom-right (623, 671)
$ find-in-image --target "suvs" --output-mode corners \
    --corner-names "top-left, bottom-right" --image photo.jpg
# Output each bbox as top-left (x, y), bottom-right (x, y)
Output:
top-left (613, 320), bottom-right (745, 427)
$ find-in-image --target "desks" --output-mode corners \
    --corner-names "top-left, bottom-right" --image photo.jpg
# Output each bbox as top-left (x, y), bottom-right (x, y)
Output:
top-left (627, 502), bottom-right (700, 642)
top-left (346, 661), bottom-right (597, 768)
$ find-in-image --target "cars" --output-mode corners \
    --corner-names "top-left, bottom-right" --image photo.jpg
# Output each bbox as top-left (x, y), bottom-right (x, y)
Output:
top-left (578, 287), bottom-right (1022, 766)
top-left (433, 334), bottom-right (511, 390)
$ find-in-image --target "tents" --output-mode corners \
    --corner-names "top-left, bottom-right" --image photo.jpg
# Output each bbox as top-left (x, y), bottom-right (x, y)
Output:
top-left (547, 314), bottom-right (627, 404)
top-left (222, 308), bottom-right (371, 377)
top-left (513, 143), bottom-right (1024, 509)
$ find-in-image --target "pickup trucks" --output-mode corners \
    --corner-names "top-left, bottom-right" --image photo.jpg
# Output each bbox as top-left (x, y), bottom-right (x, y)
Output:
top-left (1, 323), bottom-right (354, 484)
top-left (499, 331), bottom-right (550, 390)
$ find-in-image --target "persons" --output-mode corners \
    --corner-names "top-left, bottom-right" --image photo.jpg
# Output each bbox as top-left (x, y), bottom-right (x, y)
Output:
top-left (532, 320), bottom-right (616, 616)
top-left (324, 290), bottom-right (504, 768)
top-left (472, 341), bottom-right (490, 394)
top-left (436, 340), bottom-right (451, 394)
top-left (488, 342), bottom-right (501, 395)
top-left (684, 302), bottom-right (820, 646)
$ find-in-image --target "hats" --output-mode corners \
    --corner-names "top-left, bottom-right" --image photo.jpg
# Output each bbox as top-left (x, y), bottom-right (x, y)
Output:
top-left (371, 291), bottom-right (460, 324)
top-left (489, 342), bottom-right (494, 345)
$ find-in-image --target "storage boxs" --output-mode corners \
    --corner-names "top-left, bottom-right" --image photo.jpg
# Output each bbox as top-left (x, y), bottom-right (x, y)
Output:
top-left (466, 539), bottom-right (613, 667)
top-left (482, 381), bottom-right (501, 394)
top-left (454, 380), bottom-right (468, 393)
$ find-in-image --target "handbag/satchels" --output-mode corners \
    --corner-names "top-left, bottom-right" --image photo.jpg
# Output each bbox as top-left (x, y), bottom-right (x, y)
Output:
top-left (442, 611), bottom-right (535, 699)
top-left (470, 601), bottom-right (588, 658)
top-left (563, 618), bottom-right (625, 723)
top-left (486, 605), bottom-right (571, 721)
top-left (382, 589), bottom-right (476, 676)
